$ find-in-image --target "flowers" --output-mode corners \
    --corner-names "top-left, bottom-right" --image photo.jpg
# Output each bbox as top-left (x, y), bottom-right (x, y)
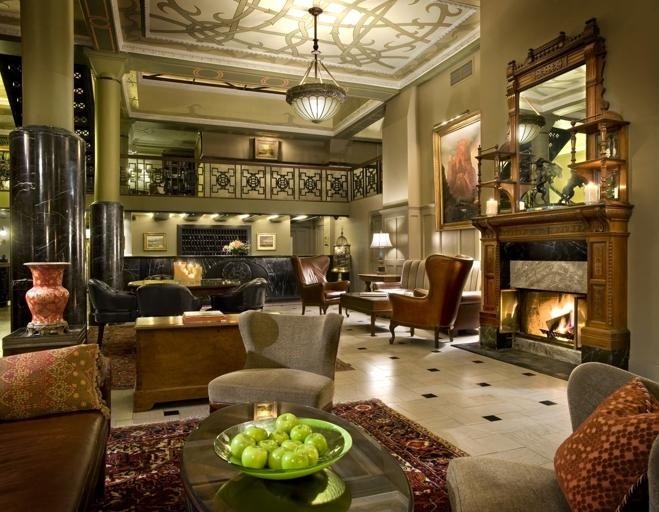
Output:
top-left (223, 240), bottom-right (250, 254)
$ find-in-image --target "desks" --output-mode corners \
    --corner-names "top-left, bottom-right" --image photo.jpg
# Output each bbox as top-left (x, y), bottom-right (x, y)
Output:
top-left (132, 312), bottom-right (246, 413)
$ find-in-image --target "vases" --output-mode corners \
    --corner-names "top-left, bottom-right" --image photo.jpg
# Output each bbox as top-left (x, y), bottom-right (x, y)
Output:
top-left (23, 262), bottom-right (72, 336)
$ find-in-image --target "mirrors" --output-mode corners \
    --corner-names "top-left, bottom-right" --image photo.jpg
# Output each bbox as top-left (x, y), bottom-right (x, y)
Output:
top-left (505, 18), bottom-right (609, 212)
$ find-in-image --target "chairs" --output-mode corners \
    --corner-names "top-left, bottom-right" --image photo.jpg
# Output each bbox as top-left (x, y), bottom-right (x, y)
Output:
top-left (446, 362), bottom-right (659, 512)
top-left (88, 277), bottom-right (268, 347)
top-left (291, 254), bottom-right (474, 348)
top-left (208, 310), bottom-right (344, 415)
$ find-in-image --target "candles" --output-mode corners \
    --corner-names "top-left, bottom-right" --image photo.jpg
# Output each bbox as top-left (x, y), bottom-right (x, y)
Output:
top-left (584, 180), bottom-right (601, 207)
top-left (518, 201), bottom-right (525, 212)
top-left (486, 197), bottom-right (498, 217)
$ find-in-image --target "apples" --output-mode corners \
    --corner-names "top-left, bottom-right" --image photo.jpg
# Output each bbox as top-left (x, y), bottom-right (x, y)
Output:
top-left (231, 412), bottom-right (328, 470)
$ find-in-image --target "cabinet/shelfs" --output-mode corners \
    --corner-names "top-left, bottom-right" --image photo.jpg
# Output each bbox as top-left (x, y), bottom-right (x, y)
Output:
top-left (475, 145), bottom-right (515, 217)
top-left (518, 152), bottom-right (537, 211)
top-left (568, 113), bottom-right (630, 207)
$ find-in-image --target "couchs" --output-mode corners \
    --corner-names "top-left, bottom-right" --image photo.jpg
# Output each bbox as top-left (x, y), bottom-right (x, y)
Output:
top-left (0, 342), bottom-right (112, 511)
top-left (369, 259), bottom-right (482, 337)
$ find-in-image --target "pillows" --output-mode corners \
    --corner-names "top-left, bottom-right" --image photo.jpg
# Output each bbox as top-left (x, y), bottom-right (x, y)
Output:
top-left (554, 376), bottom-right (659, 512)
top-left (0, 343), bottom-right (111, 421)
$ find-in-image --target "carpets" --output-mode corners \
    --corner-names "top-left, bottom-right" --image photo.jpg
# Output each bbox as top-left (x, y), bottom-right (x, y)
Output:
top-left (93, 399), bottom-right (469, 512)
top-left (84, 325), bottom-right (356, 390)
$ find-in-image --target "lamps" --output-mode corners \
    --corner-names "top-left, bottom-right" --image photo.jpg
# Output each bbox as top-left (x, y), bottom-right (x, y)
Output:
top-left (285, 8), bottom-right (346, 123)
top-left (519, 93), bottom-right (545, 145)
top-left (370, 230), bottom-right (393, 274)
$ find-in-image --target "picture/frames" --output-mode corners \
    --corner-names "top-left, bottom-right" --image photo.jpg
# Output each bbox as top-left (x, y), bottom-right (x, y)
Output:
top-left (252, 137), bottom-right (281, 162)
top-left (256, 233), bottom-right (277, 251)
top-left (143, 232), bottom-right (168, 251)
top-left (432, 108), bottom-right (481, 232)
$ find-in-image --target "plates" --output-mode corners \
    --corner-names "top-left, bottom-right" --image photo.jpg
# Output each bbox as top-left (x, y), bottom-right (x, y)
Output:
top-left (215, 418), bottom-right (352, 480)
